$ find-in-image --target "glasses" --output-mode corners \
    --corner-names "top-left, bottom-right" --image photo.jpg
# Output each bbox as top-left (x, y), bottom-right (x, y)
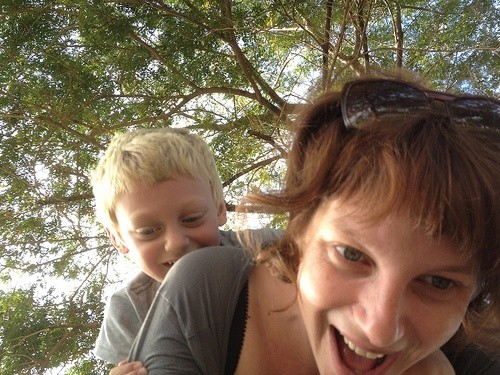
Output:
top-left (340, 78), bottom-right (500, 132)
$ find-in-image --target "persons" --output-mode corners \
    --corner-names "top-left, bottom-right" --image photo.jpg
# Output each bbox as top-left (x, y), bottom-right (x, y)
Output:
top-left (123, 66), bottom-right (499, 375)
top-left (91, 127), bottom-right (287, 375)
